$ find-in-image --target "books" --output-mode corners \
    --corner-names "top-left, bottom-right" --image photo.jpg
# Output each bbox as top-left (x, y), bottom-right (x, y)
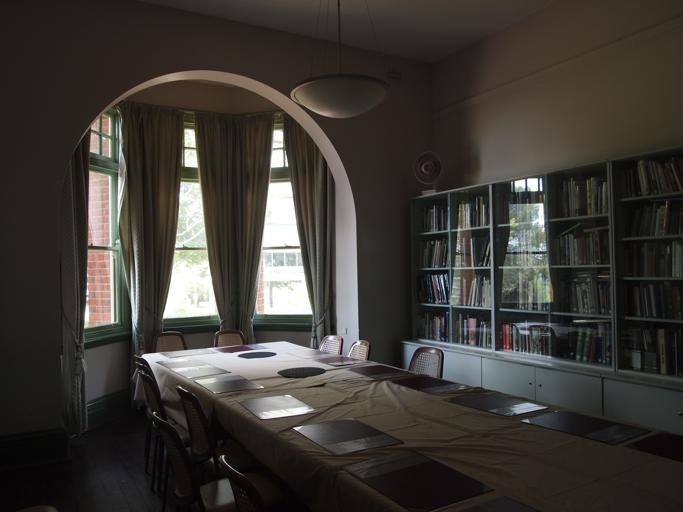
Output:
top-left (551, 225), bottom-right (610, 265)
top-left (418, 308), bottom-right (451, 343)
top-left (616, 160), bottom-right (682, 237)
top-left (454, 310), bottom-right (491, 349)
top-left (621, 323), bottom-right (682, 377)
top-left (497, 318), bottom-right (550, 355)
top-left (453, 235), bottom-right (491, 267)
top-left (499, 227), bottom-right (546, 266)
top-left (622, 239), bottom-right (682, 320)
top-left (554, 270), bottom-right (613, 316)
top-left (552, 175), bottom-right (609, 218)
top-left (497, 271), bottom-right (549, 311)
top-left (555, 319), bottom-right (613, 368)
top-left (417, 271), bottom-right (450, 305)
top-left (423, 199), bottom-right (448, 232)
top-left (419, 237), bottom-right (449, 268)
top-left (452, 271), bottom-right (491, 308)
top-left (501, 185), bottom-right (545, 224)
top-left (454, 194), bottom-right (490, 228)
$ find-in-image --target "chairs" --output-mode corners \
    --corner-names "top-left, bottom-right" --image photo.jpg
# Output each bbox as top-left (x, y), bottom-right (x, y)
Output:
top-left (318, 335), bottom-right (343, 355)
top-left (215, 330), bottom-right (246, 347)
top-left (153, 332), bottom-right (187, 353)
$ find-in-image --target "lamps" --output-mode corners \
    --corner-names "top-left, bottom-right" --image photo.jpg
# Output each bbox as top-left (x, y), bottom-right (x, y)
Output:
top-left (290, 0), bottom-right (390, 120)
